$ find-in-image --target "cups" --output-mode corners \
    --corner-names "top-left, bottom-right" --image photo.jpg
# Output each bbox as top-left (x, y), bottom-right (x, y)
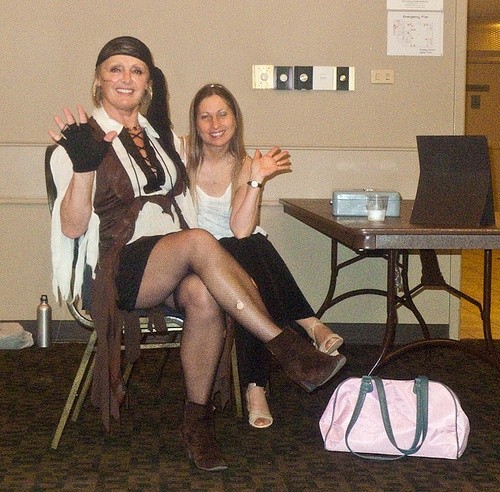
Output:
top-left (366, 194), bottom-right (389, 222)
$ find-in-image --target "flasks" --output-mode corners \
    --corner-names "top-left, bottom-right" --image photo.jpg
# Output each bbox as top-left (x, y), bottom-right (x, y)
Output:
top-left (37, 295), bottom-right (52, 348)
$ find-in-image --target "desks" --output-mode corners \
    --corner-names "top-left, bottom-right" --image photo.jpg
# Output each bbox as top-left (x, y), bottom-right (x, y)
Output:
top-left (279, 198), bottom-right (500, 381)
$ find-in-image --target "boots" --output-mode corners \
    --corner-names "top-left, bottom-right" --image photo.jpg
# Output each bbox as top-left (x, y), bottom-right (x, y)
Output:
top-left (180, 401), bottom-right (229, 471)
top-left (265, 326), bottom-right (347, 393)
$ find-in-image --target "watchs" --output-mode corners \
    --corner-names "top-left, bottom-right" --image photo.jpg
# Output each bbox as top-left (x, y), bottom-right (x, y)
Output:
top-left (247, 180), bottom-right (263, 189)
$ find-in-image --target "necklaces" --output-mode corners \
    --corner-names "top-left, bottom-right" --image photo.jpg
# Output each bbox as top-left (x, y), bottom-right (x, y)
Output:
top-left (124, 123), bottom-right (140, 131)
top-left (205, 163), bottom-right (230, 185)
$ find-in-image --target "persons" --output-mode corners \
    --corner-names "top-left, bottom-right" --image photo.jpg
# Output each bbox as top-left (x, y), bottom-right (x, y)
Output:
top-left (177, 82), bottom-right (345, 428)
top-left (50, 37), bottom-right (348, 471)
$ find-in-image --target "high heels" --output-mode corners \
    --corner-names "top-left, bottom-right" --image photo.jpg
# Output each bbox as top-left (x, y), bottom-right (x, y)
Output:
top-left (246, 384), bottom-right (274, 428)
top-left (307, 318), bottom-right (342, 357)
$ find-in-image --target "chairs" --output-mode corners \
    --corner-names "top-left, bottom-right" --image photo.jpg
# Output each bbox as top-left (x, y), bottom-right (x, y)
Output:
top-left (45, 145), bottom-right (245, 450)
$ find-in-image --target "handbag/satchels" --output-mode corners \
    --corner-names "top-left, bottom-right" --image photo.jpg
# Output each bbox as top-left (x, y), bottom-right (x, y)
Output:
top-left (319, 375), bottom-right (471, 460)
